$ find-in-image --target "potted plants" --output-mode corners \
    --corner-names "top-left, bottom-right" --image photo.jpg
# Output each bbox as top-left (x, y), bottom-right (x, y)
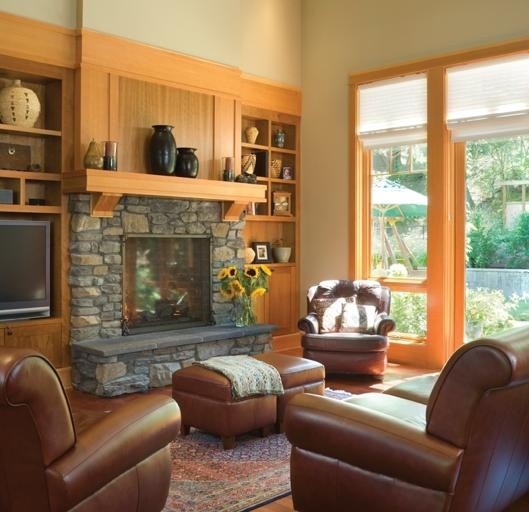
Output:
top-left (272, 237), bottom-right (295, 263)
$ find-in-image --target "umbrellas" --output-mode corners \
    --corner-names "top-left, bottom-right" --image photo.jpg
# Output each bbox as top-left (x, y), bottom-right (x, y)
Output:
top-left (370, 169), bottom-right (429, 269)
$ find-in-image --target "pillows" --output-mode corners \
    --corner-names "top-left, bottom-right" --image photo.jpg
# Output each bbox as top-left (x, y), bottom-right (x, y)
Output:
top-left (315, 293), bottom-right (381, 334)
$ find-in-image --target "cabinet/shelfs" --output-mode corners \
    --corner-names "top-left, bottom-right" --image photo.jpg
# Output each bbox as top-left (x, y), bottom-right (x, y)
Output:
top-left (240, 106), bottom-right (304, 335)
top-left (0, 54), bottom-right (77, 396)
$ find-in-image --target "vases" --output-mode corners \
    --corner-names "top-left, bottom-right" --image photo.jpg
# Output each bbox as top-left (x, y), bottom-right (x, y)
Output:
top-left (228, 298), bottom-right (254, 328)
top-left (149, 122), bottom-right (199, 178)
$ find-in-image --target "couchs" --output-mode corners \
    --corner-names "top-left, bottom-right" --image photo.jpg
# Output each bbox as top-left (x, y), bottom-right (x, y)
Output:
top-left (0, 345), bottom-right (183, 512)
top-left (283, 326), bottom-right (529, 511)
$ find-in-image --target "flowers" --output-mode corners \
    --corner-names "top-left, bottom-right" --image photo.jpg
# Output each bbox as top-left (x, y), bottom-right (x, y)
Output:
top-left (216, 261), bottom-right (273, 321)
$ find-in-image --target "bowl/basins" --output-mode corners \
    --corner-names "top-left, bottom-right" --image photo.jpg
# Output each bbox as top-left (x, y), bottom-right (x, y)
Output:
top-left (272, 247), bottom-right (292, 263)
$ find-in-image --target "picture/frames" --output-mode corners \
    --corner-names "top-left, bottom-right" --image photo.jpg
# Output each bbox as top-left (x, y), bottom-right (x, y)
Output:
top-left (250, 241), bottom-right (275, 265)
top-left (272, 190), bottom-right (294, 217)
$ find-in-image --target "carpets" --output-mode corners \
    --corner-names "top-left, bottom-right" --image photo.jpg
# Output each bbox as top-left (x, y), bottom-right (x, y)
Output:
top-left (159, 384), bottom-right (360, 512)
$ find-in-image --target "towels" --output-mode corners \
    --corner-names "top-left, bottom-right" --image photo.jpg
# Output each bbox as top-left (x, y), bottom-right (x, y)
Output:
top-left (192, 352), bottom-right (288, 400)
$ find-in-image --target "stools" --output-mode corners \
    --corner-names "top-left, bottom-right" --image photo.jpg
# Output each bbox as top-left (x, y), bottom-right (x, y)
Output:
top-left (171, 345), bottom-right (331, 452)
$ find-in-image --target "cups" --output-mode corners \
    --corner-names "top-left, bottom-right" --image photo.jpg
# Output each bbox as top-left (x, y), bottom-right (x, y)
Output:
top-left (103, 140), bottom-right (118, 171)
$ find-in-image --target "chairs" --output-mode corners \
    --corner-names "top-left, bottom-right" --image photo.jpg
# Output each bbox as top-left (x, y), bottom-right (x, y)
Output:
top-left (297, 277), bottom-right (396, 377)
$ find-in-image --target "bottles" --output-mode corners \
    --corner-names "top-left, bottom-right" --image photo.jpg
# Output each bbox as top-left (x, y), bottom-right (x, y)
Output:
top-left (222, 158), bottom-right (234, 182)
top-left (274, 128), bottom-right (288, 149)
top-left (150, 124), bottom-right (178, 175)
top-left (175, 147), bottom-right (199, 178)
top-left (83, 139), bottom-right (103, 169)
top-left (244, 126), bottom-right (259, 144)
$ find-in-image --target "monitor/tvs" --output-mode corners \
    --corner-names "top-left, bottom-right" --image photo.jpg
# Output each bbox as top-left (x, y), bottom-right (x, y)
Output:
top-left (0, 220), bottom-right (50, 322)
